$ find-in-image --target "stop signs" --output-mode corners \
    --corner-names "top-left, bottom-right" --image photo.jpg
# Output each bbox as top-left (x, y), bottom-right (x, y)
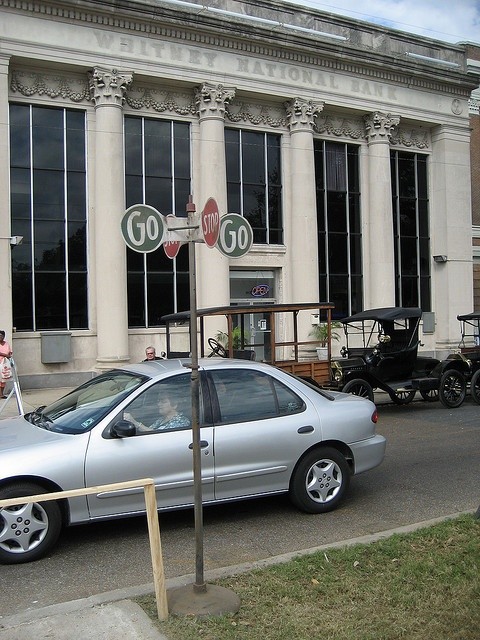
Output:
top-left (165, 216), bottom-right (182, 258)
top-left (202, 200), bottom-right (220, 246)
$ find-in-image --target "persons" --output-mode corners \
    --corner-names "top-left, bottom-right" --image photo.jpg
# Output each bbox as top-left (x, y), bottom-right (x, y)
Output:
top-left (123, 389), bottom-right (190, 432)
top-left (0, 331), bottom-right (13, 399)
top-left (139, 347), bottom-right (163, 363)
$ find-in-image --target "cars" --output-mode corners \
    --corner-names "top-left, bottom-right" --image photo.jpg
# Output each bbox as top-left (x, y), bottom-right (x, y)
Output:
top-left (0, 358), bottom-right (385, 562)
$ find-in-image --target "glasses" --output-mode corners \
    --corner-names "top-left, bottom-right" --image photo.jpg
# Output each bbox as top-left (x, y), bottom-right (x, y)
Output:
top-left (156, 399), bottom-right (167, 403)
top-left (147, 353), bottom-right (154, 355)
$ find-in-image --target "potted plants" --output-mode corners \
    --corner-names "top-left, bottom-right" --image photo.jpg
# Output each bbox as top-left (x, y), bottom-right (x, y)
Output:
top-left (308, 321), bottom-right (343, 361)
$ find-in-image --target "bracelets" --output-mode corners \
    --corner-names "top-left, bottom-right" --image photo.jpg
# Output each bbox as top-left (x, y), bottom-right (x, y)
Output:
top-left (136, 423), bottom-right (143, 430)
